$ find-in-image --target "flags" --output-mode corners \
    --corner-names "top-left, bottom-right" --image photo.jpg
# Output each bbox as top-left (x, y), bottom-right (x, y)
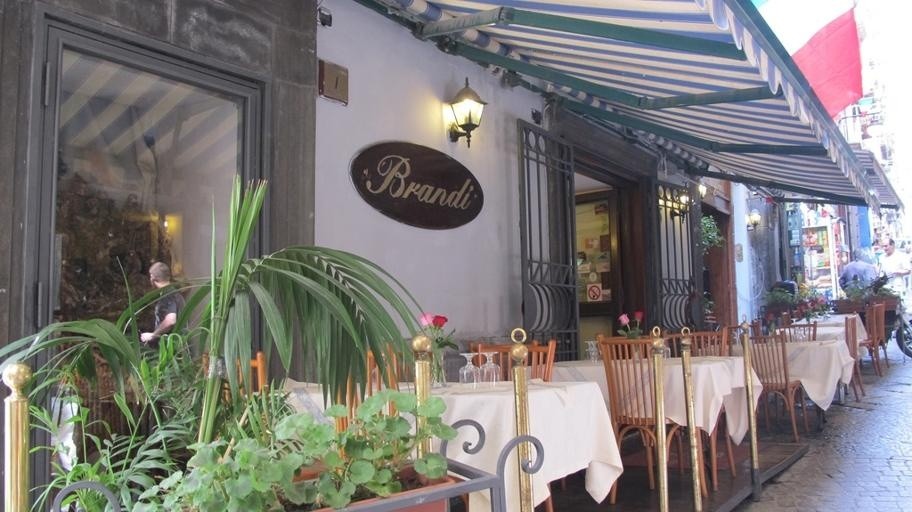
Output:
top-left (790, 7), bottom-right (863, 118)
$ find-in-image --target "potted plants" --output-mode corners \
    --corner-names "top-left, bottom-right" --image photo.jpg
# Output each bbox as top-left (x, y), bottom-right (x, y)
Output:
top-left (1, 173), bottom-right (499, 512)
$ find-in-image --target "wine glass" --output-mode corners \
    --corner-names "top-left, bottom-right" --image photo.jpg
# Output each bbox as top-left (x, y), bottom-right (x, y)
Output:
top-left (583, 340), bottom-right (600, 366)
top-left (458, 351), bottom-right (481, 395)
top-left (478, 351), bottom-right (501, 394)
top-left (789, 318), bottom-right (805, 343)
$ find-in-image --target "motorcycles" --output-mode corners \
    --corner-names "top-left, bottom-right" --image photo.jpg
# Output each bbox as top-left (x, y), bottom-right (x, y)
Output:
top-left (771, 260), bottom-right (912, 357)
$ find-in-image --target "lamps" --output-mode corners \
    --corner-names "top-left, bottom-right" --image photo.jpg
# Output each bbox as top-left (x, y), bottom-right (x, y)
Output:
top-left (747, 209), bottom-right (761, 232)
top-left (448, 77), bottom-right (488, 148)
top-left (671, 191), bottom-right (690, 223)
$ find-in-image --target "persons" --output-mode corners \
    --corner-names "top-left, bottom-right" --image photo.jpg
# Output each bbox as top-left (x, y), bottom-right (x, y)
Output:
top-left (838, 228), bottom-right (912, 355)
top-left (139, 261), bottom-right (188, 369)
